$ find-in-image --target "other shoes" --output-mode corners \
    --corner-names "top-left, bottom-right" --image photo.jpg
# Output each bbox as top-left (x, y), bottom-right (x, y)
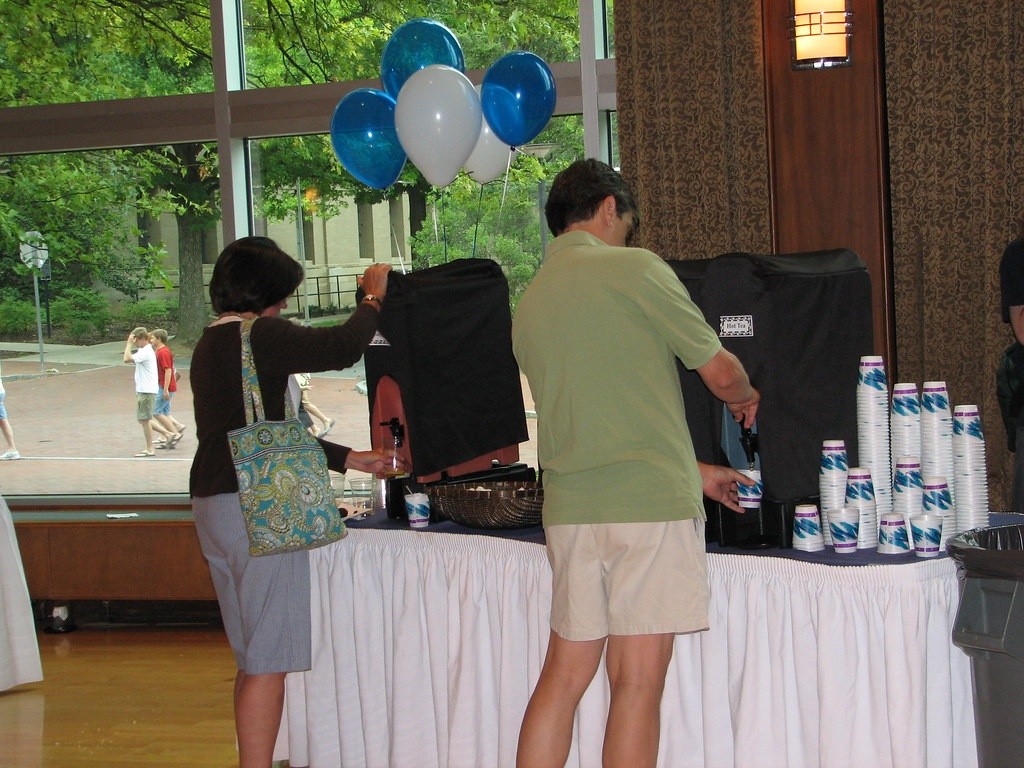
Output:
top-left (135, 424), bottom-right (186, 456)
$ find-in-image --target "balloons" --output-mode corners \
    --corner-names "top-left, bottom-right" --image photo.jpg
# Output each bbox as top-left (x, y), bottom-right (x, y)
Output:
top-left (330, 87), bottom-right (407, 190)
top-left (394, 65), bottom-right (482, 187)
top-left (480, 50), bottom-right (556, 151)
top-left (381, 17), bottom-right (464, 102)
top-left (462, 83), bottom-right (525, 184)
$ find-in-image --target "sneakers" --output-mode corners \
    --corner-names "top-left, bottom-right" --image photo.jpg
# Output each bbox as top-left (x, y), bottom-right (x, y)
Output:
top-left (0, 451), bottom-right (20, 461)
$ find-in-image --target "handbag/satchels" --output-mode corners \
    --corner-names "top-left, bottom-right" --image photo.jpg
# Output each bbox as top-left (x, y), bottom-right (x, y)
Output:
top-left (175, 369), bottom-right (181, 382)
top-left (226, 419), bottom-right (350, 558)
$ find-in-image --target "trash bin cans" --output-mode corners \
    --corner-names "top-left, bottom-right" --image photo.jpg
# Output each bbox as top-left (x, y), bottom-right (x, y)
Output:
top-left (945, 523), bottom-right (1024, 768)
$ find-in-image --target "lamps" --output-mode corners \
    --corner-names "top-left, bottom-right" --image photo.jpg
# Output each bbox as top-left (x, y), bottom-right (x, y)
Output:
top-left (787, 1), bottom-right (856, 70)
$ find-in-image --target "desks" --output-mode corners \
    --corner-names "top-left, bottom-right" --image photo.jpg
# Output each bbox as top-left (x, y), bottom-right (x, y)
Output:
top-left (268, 510), bottom-right (984, 768)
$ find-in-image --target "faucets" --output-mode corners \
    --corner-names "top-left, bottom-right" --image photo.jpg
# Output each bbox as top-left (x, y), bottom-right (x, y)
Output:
top-left (733, 413), bottom-right (755, 467)
top-left (380, 417), bottom-right (403, 448)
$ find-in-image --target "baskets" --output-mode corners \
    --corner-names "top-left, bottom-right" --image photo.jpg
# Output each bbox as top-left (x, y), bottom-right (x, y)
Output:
top-left (427, 480), bottom-right (544, 528)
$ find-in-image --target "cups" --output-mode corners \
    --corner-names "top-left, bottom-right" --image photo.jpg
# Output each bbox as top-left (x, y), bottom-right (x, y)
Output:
top-left (406, 494), bottom-right (433, 527)
top-left (734, 469), bottom-right (765, 509)
top-left (381, 436), bottom-right (406, 475)
top-left (329, 473), bottom-right (386, 520)
top-left (791, 355), bottom-right (990, 557)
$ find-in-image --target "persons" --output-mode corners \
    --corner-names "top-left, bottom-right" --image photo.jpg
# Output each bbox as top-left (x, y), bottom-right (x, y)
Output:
top-left (994, 238), bottom-right (1024, 452)
top-left (287, 318), bottom-right (335, 438)
top-left (516, 157), bottom-right (760, 768)
top-left (190, 236), bottom-right (392, 768)
top-left (147, 329), bottom-right (185, 449)
top-left (0, 378), bottom-right (20, 461)
top-left (124, 327), bottom-right (175, 458)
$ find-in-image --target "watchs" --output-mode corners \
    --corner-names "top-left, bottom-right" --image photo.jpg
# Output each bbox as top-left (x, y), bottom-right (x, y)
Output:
top-left (361, 294), bottom-right (382, 306)
